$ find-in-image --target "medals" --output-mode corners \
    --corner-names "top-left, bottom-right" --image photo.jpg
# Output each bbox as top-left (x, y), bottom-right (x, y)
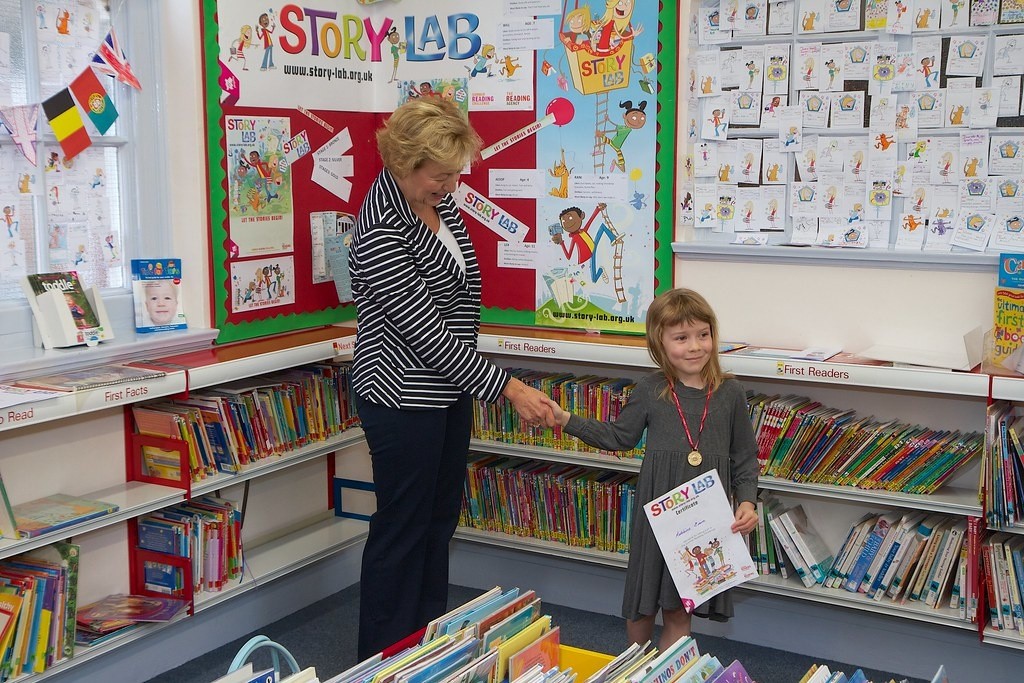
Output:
top-left (688, 447), bottom-right (702, 466)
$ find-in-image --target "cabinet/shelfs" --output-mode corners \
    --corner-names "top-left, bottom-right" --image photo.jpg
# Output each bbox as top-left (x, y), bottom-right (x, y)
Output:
top-left (0, 322), bottom-right (1024, 683)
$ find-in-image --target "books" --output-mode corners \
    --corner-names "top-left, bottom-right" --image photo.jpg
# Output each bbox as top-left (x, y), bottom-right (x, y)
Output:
top-left (991, 252), bottom-right (1024, 377)
top-left (0, 341), bottom-right (1024, 683)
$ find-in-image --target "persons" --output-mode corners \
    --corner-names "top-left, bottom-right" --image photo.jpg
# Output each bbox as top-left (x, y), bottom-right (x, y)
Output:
top-left (143, 280), bottom-right (178, 326)
top-left (64, 294), bottom-right (89, 326)
top-left (540, 287), bottom-right (758, 661)
top-left (347, 96), bottom-right (557, 665)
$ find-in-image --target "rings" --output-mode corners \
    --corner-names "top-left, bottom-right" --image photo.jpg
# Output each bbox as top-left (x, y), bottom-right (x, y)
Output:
top-left (534, 422), bottom-right (540, 429)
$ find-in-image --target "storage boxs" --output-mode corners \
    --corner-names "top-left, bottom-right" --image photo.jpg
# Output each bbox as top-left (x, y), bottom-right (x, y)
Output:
top-left (980, 327), bottom-right (1023, 376)
